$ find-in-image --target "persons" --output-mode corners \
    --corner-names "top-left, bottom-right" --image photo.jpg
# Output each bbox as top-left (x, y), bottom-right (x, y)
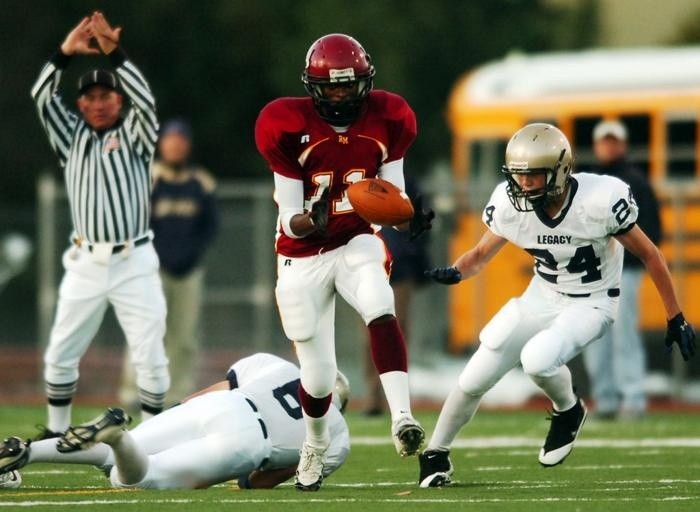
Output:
top-left (0, 351), bottom-right (351, 491)
top-left (419, 123), bottom-right (699, 488)
top-left (30, 11), bottom-right (170, 442)
top-left (254, 34), bottom-right (425, 492)
top-left (117, 120), bottom-right (217, 409)
top-left (581, 119), bottom-right (661, 417)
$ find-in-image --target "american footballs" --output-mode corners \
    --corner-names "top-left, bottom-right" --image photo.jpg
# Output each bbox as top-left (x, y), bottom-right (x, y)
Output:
top-left (344, 177), bottom-right (416, 226)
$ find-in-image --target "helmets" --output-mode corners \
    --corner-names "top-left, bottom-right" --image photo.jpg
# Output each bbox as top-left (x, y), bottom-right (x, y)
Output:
top-left (334, 370), bottom-right (350, 413)
top-left (299, 33), bottom-right (373, 123)
top-left (499, 122), bottom-right (576, 214)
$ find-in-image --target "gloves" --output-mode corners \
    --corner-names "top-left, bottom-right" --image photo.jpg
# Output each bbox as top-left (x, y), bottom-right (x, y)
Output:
top-left (307, 187), bottom-right (334, 232)
top-left (416, 265), bottom-right (462, 287)
top-left (407, 192), bottom-right (437, 244)
top-left (662, 313), bottom-right (700, 361)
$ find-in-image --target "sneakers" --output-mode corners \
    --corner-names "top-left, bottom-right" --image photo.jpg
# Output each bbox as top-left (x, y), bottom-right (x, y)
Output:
top-left (0, 435), bottom-right (30, 479)
top-left (538, 397), bottom-right (589, 468)
top-left (54, 405), bottom-right (132, 454)
top-left (294, 435), bottom-right (332, 494)
top-left (31, 429), bottom-right (65, 441)
top-left (389, 415), bottom-right (427, 461)
top-left (415, 448), bottom-right (455, 489)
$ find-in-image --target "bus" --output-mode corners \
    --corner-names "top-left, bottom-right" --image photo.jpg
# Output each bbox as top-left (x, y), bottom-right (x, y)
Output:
top-left (444, 48), bottom-right (700, 359)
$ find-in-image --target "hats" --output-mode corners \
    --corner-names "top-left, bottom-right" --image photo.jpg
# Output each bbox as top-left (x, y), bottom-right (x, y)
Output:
top-left (592, 118), bottom-right (630, 142)
top-left (161, 118), bottom-right (193, 134)
top-left (75, 68), bottom-right (124, 96)
